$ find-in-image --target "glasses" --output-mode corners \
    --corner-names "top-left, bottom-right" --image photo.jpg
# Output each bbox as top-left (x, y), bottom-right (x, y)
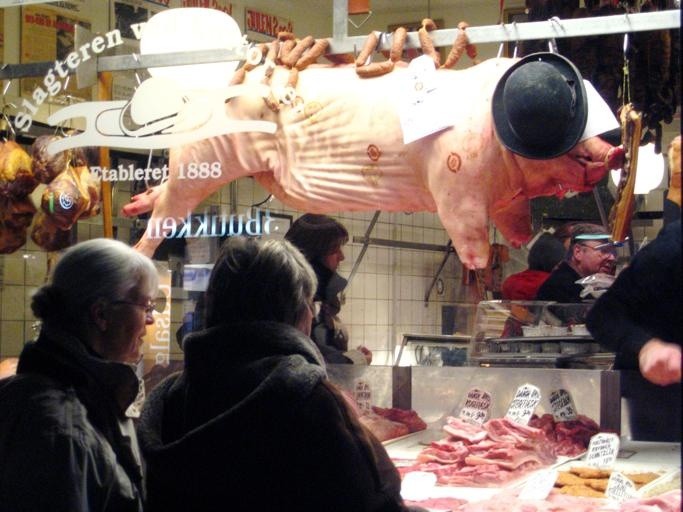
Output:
top-left (102, 300), bottom-right (157, 314)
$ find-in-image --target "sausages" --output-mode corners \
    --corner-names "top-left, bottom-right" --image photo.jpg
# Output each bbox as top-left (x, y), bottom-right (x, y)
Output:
top-left (463, 242), bottom-right (509, 307)
top-left (230, 17), bottom-right (477, 86)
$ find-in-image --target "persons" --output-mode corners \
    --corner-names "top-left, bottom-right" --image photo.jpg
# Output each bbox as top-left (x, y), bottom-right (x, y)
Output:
top-left (585, 216), bottom-right (683, 442)
top-left (553, 221), bottom-right (583, 249)
top-left (0, 238), bottom-right (159, 512)
top-left (137, 233), bottom-right (424, 512)
top-left (535, 223), bottom-right (617, 327)
top-left (500, 236), bottom-right (567, 338)
top-left (284, 214), bottom-right (372, 386)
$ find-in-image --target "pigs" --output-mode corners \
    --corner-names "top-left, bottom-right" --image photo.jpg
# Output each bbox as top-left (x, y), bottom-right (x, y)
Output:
top-left (122, 62), bottom-right (629, 272)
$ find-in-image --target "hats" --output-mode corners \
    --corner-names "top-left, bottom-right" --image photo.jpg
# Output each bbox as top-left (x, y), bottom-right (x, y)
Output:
top-left (490, 50), bottom-right (588, 160)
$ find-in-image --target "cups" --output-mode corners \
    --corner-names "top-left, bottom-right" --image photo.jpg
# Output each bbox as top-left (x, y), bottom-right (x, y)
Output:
top-left (521, 323), bottom-right (585, 338)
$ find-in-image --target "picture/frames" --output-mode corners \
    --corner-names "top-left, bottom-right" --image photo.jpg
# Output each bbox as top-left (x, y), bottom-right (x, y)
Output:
top-left (258, 211), bottom-right (293, 241)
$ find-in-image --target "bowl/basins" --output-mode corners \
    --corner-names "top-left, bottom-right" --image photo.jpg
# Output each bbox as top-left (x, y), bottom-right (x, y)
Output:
top-left (476, 342), bottom-right (599, 358)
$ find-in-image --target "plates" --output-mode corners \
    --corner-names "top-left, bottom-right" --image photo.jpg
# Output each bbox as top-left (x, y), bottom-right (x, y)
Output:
top-left (572, 274), bottom-right (608, 299)
